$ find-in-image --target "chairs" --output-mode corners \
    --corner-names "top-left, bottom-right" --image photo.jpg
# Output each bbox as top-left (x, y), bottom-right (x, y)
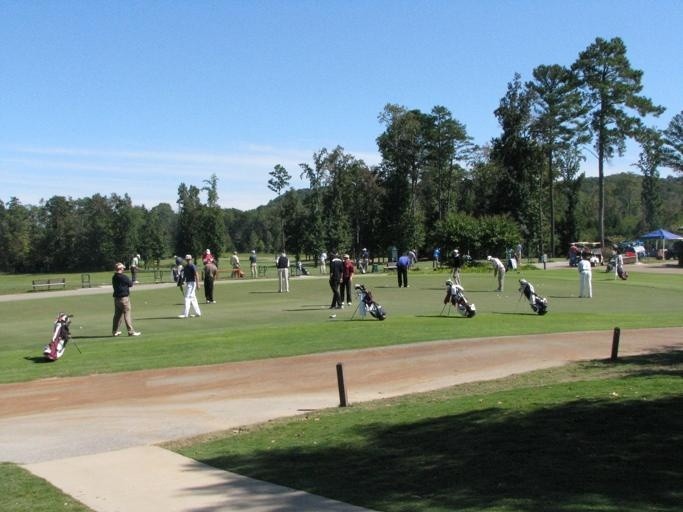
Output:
top-left (80, 273), bottom-right (90, 288)
top-left (31, 277), bottom-right (64, 290)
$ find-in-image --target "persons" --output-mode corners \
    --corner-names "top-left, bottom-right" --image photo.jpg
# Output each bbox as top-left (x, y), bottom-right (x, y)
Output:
top-left (174, 255), bottom-right (183, 273)
top-left (320, 248), bottom-right (354, 309)
top-left (112, 263), bottom-right (141, 337)
top-left (452, 249), bottom-right (462, 286)
top-left (276, 252), bottom-right (290, 292)
top-left (202, 249), bottom-right (213, 265)
top-left (130, 254), bottom-right (141, 283)
top-left (361, 248), bottom-right (369, 271)
top-left (578, 259), bottom-right (593, 298)
top-left (230, 251), bottom-right (240, 277)
top-left (201, 260), bottom-right (217, 304)
top-left (432, 247), bottom-right (441, 269)
top-left (174, 254), bottom-right (201, 318)
top-left (487, 256), bottom-right (505, 292)
top-left (396, 256), bottom-right (411, 288)
top-left (568, 243), bottom-right (578, 266)
top-left (249, 250), bottom-right (257, 279)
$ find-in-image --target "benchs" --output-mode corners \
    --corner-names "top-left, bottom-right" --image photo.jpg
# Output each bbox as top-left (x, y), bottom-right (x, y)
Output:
top-left (383, 265), bottom-right (398, 273)
top-left (216, 269), bottom-right (240, 278)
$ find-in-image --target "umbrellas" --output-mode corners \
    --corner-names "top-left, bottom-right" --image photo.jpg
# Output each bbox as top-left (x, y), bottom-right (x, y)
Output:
top-left (640, 229), bottom-right (683, 261)
